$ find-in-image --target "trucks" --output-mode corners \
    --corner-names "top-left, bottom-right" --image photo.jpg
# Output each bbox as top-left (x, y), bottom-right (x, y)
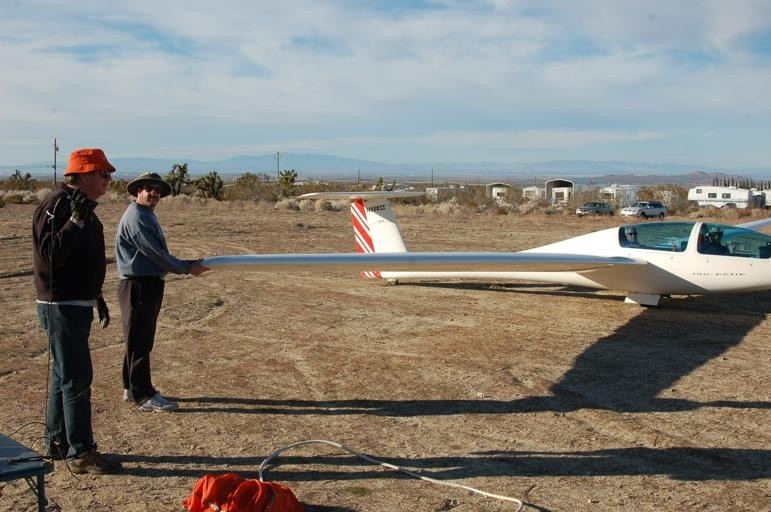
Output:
top-left (688, 186), bottom-right (771, 212)
top-left (600, 184), bottom-right (643, 208)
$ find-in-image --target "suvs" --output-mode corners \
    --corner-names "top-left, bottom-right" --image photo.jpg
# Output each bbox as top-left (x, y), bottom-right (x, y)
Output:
top-left (577, 201), bottom-right (617, 218)
top-left (621, 200), bottom-right (666, 218)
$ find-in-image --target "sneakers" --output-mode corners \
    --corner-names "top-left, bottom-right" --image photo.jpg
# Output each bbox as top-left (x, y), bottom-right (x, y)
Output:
top-left (122, 388), bottom-right (134, 402)
top-left (136, 390), bottom-right (179, 413)
top-left (68, 441), bottom-right (124, 474)
top-left (47, 443), bottom-right (71, 461)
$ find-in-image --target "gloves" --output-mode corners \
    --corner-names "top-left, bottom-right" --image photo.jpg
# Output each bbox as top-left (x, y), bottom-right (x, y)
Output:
top-left (95, 295), bottom-right (110, 330)
top-left (69, 187), bottom-right (99, 224)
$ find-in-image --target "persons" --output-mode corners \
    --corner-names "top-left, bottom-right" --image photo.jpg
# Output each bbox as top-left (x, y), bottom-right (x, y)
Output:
top-left (704, 226), bottom-right (731, 255)
top-left (114, 172), bottom-right (211, 413)
top-left (622, 225), bottom-right (648, 249)
top-left (30, 147), bottom-right (126, 476)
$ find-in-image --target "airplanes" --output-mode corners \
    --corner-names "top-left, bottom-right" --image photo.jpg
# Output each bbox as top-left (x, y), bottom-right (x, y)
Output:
top-left (197, 190), bottom-right (771, 310)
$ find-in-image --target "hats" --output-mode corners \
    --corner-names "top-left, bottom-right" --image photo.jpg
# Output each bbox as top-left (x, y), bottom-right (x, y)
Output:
top-left (63, 148), bottom-right (116, 176)
top-left (126, 170), bottom-right (172, 199)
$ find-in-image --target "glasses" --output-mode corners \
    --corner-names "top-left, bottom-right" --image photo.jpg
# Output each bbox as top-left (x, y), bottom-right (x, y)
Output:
top-left (711, 232), bottom-right (721, 237)
top-left (88, 170), bottom-right (110, 178)
top-left (145, 185), bottom-right (161, 193)
